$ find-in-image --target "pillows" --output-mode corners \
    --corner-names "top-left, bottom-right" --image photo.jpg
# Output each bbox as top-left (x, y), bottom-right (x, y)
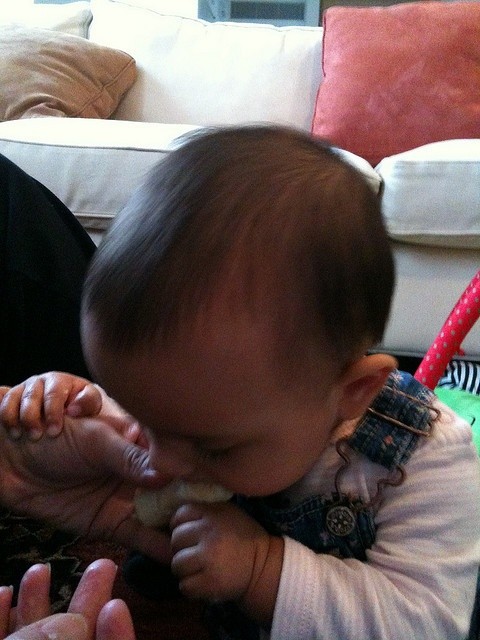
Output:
top-left (310, 0), bottom-right (480, 168)
top-left (4, 7), bottom-right (93, 39)
top-left (1, 23), bottom-right (136, 122)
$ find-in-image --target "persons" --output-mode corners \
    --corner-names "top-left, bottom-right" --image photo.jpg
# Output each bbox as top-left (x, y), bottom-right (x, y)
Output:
top-left (0, 154), bottom-right (228, 640)
top-left (1, 123), bottom-right (480, 640)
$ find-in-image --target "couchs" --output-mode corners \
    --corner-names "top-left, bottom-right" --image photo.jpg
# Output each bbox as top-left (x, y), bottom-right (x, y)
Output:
top-left (1, 0), bottom-right (479, 363)
